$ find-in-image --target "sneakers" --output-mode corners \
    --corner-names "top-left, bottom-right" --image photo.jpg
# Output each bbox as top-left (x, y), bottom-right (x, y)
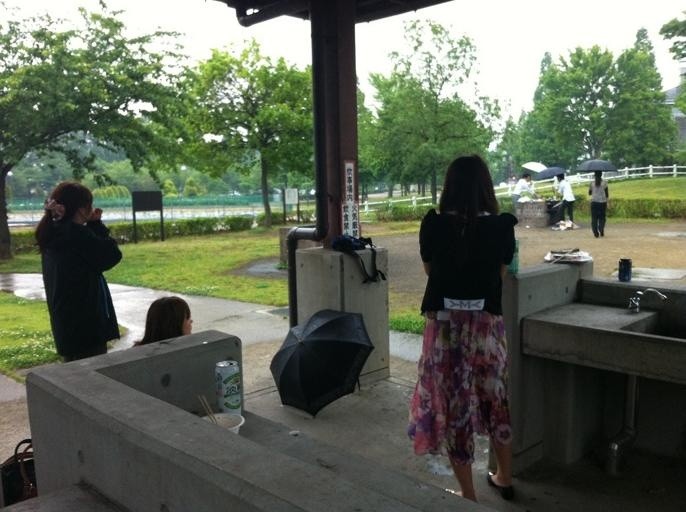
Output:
top-left (487, 470), bottom-right (516, 502)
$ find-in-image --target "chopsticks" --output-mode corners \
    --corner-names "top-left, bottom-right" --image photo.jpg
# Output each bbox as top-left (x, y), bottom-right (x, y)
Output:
top-left (197, 394), bottom-right (217, 424)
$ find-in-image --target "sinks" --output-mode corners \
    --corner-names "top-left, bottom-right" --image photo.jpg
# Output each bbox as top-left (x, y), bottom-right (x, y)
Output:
top-left (620, 312), bottom-right (686, 342)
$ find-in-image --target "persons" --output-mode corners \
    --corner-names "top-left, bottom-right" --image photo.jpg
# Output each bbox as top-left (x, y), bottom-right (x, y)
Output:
top-left (512, 173), bottom-right (540, 225)
top-left (589, 170), bottom-right (611, 238)
top-left (33, 182), bottom-right (121, 360)
top-left (406, 153), bottom-right (520, 503)
top-left (129, 296), bottom-right (193, 347)
top-left (552, 173), bottom-right (576, 229)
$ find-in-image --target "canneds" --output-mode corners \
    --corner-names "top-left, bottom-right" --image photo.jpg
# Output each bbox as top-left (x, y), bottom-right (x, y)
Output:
top-left (618, 258), bottom-right (631, 281)
top-left (214, 361), bottom-right (243, 417)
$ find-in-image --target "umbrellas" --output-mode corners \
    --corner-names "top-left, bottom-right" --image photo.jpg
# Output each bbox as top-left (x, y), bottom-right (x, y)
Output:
top-left (521, 160), bottom-right (547, 174)
top-left (577, 158), bottom-right (619, 172)
top-left (269, 309), bottom-right (375, 417)
top-left (535, 164), bottom-right (567, 181)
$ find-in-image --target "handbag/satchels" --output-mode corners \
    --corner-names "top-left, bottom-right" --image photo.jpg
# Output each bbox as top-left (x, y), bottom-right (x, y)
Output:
top-left (331, 234), bottom-right (381, 283)
top-left (1, 439), bottom-right (38, 506)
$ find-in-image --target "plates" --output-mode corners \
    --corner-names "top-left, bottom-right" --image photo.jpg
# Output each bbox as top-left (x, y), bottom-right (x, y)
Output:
top-left (544, 253), bottom-right (593, 264)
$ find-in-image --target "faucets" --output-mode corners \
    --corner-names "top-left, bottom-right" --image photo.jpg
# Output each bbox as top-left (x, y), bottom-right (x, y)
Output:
top-left (639, 289), bottom-right (667, 301)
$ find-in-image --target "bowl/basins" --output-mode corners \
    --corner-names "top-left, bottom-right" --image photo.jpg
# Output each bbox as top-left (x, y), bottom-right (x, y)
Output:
top-left (200, 412), bottom-right (245, 435)
